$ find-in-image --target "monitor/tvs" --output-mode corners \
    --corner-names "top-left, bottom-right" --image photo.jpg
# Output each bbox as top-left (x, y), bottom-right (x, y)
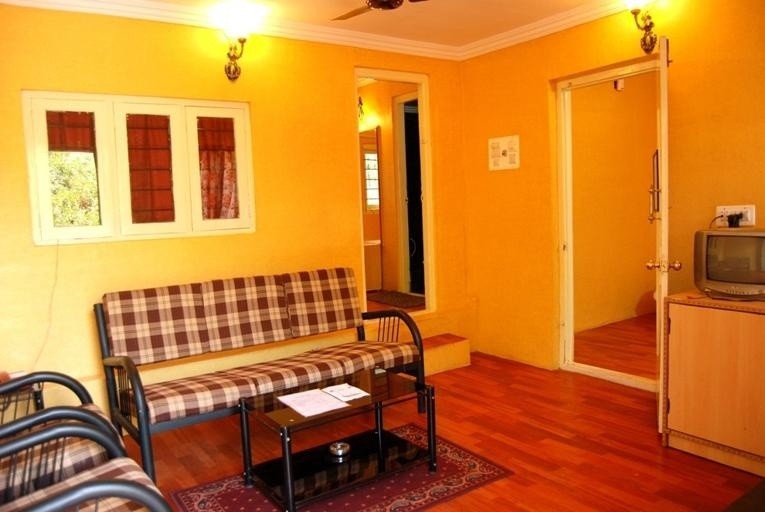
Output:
top-left (694, 231), bottom-right (765, 301)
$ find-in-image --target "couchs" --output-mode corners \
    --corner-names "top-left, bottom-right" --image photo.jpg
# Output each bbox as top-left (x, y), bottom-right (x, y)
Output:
top-left (93, 268), bottom-right (425, 485)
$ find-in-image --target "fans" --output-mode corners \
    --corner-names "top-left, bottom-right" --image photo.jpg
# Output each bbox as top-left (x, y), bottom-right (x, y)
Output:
top-left (333, 0), bottom-right (429, 21)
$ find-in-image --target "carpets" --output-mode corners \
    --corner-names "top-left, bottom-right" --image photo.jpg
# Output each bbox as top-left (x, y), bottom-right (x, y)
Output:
top-left (726, 477), bottom-right (765, 511)
top-left (367, 289), bottom-right (425, 309)
top-left (167, 422), bottom-right (514, 512)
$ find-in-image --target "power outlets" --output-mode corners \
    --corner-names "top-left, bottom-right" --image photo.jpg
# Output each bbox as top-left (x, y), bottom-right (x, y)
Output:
top-left (716, 205), bottom-right (755, 227)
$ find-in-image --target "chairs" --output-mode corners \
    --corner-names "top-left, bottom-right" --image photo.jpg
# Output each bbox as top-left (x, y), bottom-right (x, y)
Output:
top-left (0, 369), bottom-right (127, 500)
top-left (0, 424), bottom-right (171, 512)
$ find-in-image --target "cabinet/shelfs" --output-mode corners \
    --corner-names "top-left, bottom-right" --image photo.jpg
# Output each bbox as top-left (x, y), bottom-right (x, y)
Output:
top-left (665, 286), bottom-right (765, 476)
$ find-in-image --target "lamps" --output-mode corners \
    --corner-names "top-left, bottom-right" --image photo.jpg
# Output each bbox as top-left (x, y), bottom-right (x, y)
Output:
top-left (624, 0), bottom-right (656, 52)
top-left (209, 0), bottom-right (271, 79)
